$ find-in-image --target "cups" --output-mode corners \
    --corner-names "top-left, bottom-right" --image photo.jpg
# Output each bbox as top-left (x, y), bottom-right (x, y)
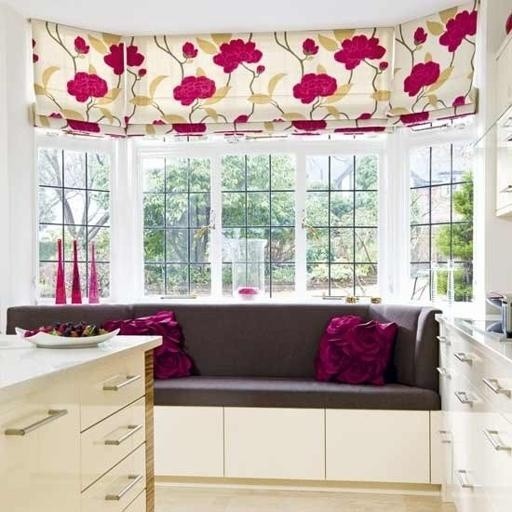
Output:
top-left (500, 293), bottom-right (512, 339)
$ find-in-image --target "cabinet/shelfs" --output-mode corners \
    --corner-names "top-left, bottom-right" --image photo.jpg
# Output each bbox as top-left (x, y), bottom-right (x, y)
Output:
top-left (495, 36), bottom-right (512, 216)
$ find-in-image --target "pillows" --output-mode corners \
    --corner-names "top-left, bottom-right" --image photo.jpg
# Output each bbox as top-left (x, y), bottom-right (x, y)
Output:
top-left (314, 312), bottom-right (396, 386)
top-left (100, 310), bottom-right (192, 379)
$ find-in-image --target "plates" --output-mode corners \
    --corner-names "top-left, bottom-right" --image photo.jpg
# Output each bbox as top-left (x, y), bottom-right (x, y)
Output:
top-left (13, 326), bottom-right (122, 350)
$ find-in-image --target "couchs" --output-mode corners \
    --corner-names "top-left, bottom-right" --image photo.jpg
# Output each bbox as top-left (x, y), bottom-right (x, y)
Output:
top-left (6, 304), bottom-right (442, 492)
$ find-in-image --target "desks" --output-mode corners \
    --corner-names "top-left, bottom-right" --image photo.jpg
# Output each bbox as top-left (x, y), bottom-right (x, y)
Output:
top-left (433, 312), bottom-right (511, 512)
top-left (1, 335), bottom-right (164, 512)
